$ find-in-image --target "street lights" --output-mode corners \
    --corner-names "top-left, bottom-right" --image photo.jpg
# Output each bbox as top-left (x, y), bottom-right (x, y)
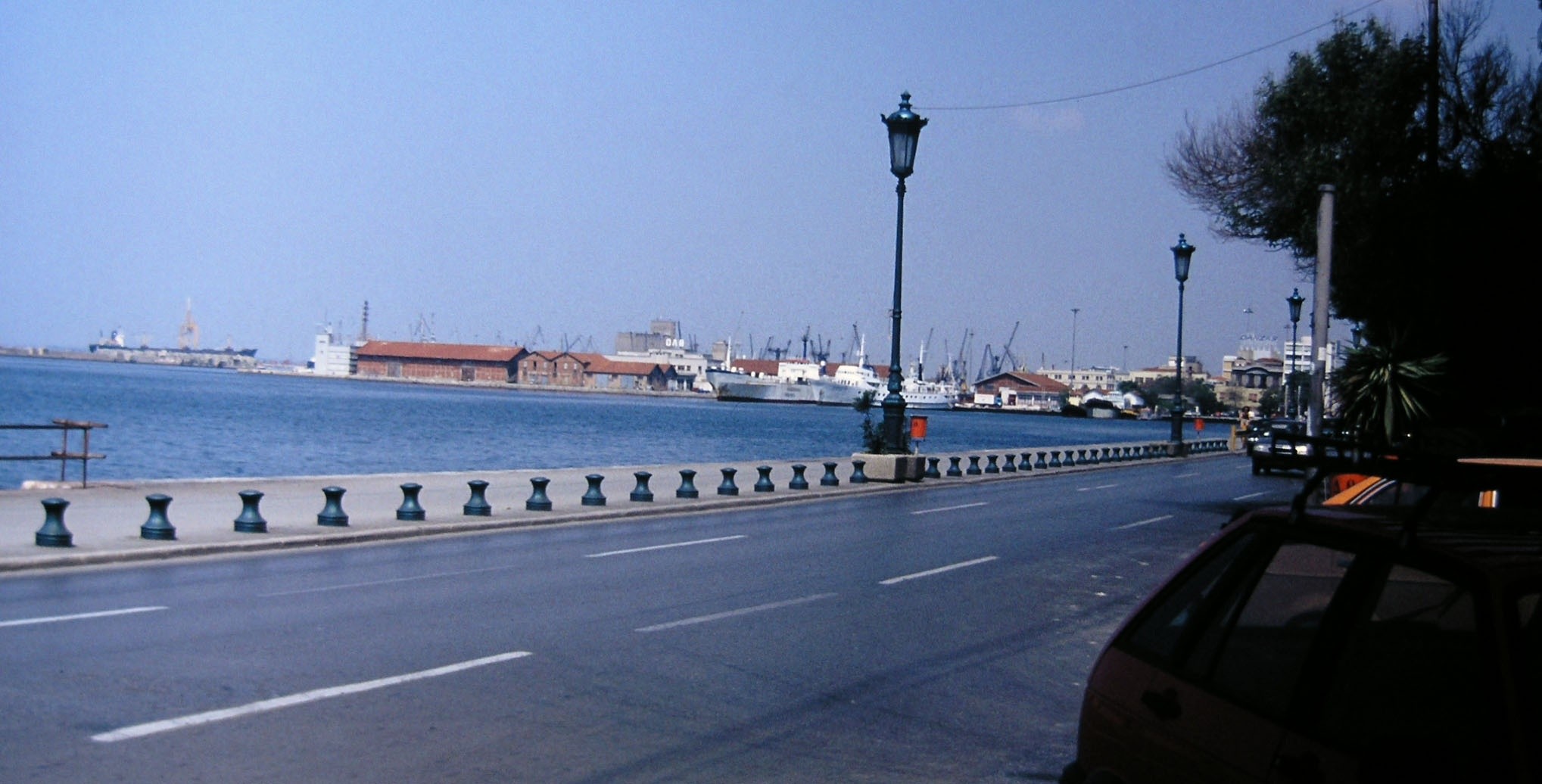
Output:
top-left (1168, 231), bottom-right (1196, 444)
top-left (1069, 309), bottom-right (1080, 387)
top-left (1285, 288), bottom-right (1305, 419)
top-left (879, 92), bottom-right (928, 453)
top-left (1123, 345), bottom-right (1128, 370)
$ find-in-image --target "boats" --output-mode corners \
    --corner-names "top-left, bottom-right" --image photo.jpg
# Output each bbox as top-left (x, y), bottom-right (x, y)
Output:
top-left (88, 294), bottom-right (264, 368)
top-left (876, 341), bottom-right (953, 411)
top-left (705, 330), bottom-right (880, 404)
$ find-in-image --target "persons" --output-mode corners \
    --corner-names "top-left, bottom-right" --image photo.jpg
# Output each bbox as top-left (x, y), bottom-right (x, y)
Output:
top-left (1237, 405), bottom-right (1251, 448)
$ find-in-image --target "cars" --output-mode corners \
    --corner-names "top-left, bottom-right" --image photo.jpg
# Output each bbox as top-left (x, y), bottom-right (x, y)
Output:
top-left (1243, 418), bottom-right (1312, 476)
